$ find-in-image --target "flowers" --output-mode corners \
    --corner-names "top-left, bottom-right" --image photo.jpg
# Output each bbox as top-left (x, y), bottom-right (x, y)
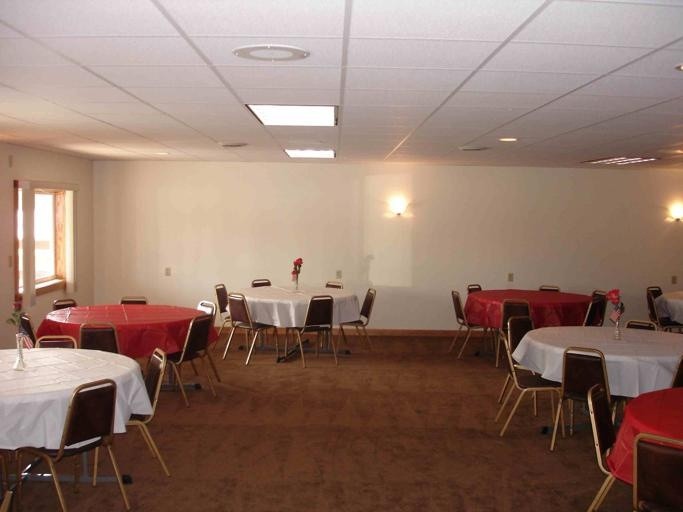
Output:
top-left (291, 258), bottom-right (303, 281)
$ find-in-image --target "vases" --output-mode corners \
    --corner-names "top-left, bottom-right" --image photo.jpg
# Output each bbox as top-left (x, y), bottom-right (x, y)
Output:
top-left (294, 271), bottom-right (299, 289)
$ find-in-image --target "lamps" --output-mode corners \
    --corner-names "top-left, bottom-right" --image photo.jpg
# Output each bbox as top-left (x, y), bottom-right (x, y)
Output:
top-left (246, 103), bottom-right (338, 128)
